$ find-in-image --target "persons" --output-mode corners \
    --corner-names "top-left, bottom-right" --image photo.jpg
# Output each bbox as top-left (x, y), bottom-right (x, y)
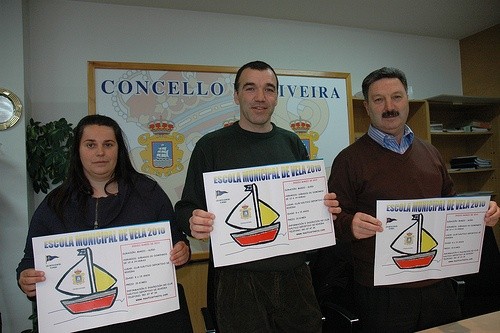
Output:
top-left (175, 61), bottom-right (341, 333)
top-left (328, 67), bottom-right (500, 333)
top-left (16, 115), bottom-right (191, 333)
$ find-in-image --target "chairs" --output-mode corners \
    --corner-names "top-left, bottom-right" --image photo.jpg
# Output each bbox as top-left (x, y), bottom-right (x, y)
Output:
top-left (32, 226), bottom-right (500, 333)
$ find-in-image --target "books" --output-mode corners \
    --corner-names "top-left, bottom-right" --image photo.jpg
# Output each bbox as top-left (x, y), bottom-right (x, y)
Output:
top-left (451, 157), bottom-right (492, 168)
top-left (430, 122), bottom-right (492, 132)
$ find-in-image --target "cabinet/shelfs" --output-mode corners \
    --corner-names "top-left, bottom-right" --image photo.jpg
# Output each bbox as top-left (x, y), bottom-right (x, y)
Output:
top-left (352, 94), bottom-right (500, 216)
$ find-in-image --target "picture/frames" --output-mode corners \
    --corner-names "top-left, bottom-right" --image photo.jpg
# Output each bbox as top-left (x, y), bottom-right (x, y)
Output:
top-left (86, 59), bottom-right (356, 264)
